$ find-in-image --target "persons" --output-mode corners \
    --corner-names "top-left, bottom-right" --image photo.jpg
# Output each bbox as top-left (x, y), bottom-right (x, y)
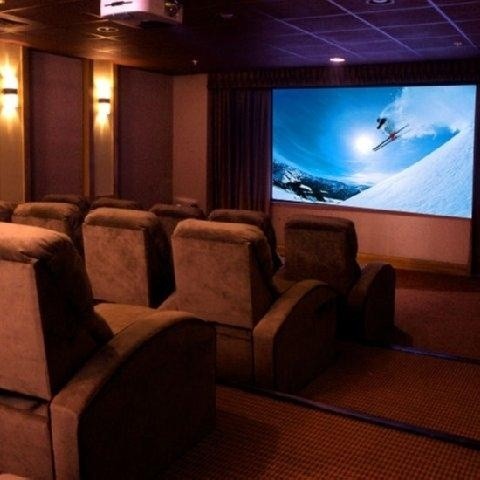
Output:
top-left (376, 117), bottom-right (398, 142)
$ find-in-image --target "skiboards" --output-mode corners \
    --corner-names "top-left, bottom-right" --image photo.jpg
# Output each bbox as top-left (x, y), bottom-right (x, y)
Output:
top-left (373, 123), bottom-right (411, 151)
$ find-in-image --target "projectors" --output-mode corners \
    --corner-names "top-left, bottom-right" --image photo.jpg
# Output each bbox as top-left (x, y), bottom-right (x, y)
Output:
top-left (100, 0), bottom-right (183, 33)
